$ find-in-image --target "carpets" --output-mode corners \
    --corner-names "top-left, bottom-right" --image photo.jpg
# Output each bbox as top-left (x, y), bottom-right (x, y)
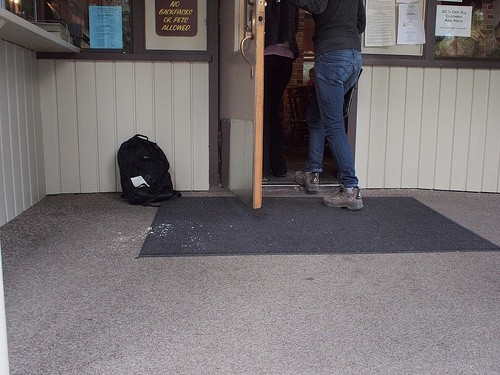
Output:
top-left (134, 196), bottom-right (500, 259)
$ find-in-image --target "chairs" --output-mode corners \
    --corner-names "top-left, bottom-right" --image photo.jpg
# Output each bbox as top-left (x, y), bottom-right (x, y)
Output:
top-left (286, 86), bottom-right (314, 156)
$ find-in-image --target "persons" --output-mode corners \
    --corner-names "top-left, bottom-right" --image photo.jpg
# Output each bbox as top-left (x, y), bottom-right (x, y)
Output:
top-left (288, 0), bottom-right (365, 210)
top-left (262, 0), bottom-right (300, 176)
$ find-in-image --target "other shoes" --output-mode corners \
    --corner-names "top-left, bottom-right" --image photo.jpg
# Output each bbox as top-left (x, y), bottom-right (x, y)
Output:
top-left (269, 162), bottom-right (287, 179)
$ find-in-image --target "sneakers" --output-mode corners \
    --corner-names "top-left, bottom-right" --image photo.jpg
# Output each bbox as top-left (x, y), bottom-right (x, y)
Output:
top-left (323, 188), bottom-right (362, 212)
top-left (293, 169), bottom-right (321, 195)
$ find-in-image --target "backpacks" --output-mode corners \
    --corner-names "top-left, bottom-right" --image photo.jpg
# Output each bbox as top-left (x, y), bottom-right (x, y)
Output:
top-left (116, 133), bottom-right (182, 205)
